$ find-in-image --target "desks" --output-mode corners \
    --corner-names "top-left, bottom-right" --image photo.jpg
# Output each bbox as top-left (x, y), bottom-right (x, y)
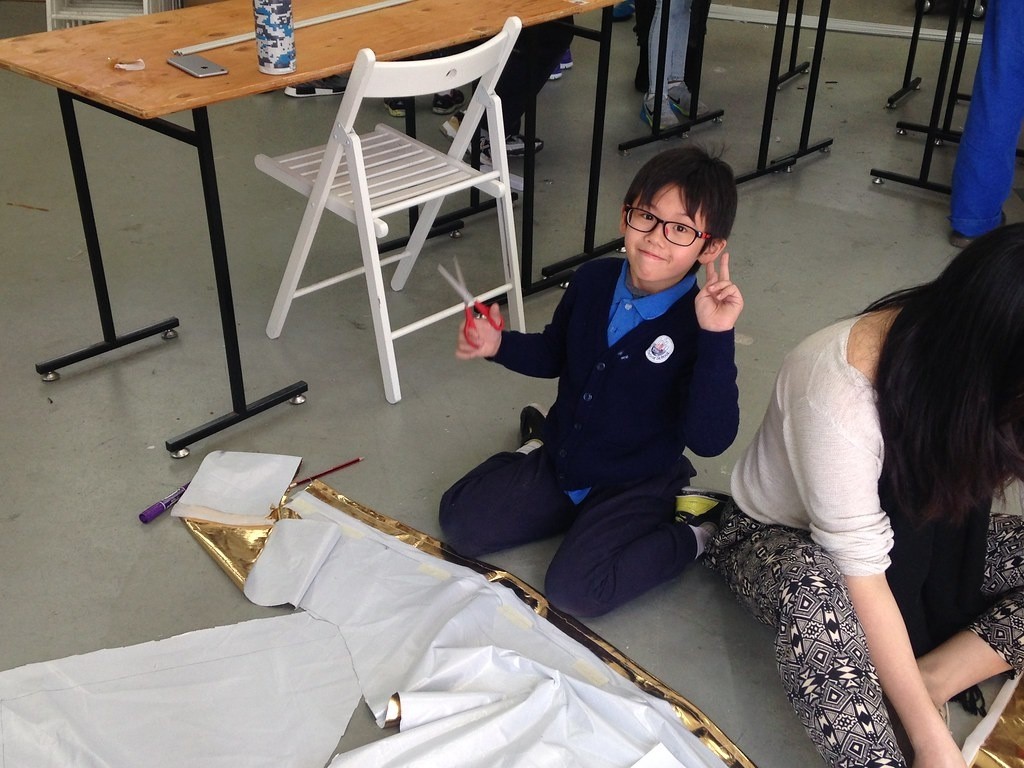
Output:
top-left (0, 0), bottom-right (1024, 460)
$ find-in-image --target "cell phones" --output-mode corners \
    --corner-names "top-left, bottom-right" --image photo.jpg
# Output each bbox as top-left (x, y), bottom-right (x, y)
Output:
top-left (167, 55), bottom-right (228, 78)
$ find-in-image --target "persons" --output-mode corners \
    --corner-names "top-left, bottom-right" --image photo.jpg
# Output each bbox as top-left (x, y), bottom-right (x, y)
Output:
top-left (380, 0), bottom-right (575, 167)
top-left (947, 0), bottom-right (1024, 247)
top-left (701, 222), bottom-right (1024, 768)
top-left (604, 0), bottom-right (709, 132)
top-left (439, 142), bottom-right (737, 620)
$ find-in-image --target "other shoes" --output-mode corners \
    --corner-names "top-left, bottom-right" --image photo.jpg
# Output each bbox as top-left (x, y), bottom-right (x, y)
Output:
top-left (548, 65), bottom-right (562, 80)
top-left (560, 47), bottom-right (573, 69)
top-left (635, 57), bottom-right (649, 91)
top-left (950, 210), bottom-right (1006, 248)
top-left (612, 0), bottom-right (636, 21)
top-left (284, 74), bottom-right (349, 98)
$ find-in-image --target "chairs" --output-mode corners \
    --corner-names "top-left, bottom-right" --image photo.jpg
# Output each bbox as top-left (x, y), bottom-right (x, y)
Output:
top-left (254, 16), bottom-right (526, 404)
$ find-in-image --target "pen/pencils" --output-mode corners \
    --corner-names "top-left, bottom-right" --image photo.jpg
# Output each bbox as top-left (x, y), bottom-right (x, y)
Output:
top-left (288, 456), bottom-right (366, 490)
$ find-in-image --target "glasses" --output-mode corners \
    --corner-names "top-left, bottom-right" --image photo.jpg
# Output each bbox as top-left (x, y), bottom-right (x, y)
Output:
top-left (624, 205), bottom-right (711, 247)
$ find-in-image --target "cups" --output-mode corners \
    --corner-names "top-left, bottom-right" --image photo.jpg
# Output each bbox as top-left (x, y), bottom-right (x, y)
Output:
top-left (252, 0), bottom-right (296, 75)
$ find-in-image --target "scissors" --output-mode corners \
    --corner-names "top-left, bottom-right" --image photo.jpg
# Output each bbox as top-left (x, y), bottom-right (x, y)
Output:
top-left (437, 256), bottom-right (506, 350)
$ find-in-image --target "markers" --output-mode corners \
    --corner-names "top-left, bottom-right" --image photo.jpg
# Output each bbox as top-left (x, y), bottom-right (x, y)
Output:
top-left (138, 480), bottom-right (191, 524)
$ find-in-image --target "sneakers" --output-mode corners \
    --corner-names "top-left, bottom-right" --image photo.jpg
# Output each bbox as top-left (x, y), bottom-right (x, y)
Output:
top-left (521, 402), bottom-right (548, 446)
top-left (675, 486), bottom-right (734, 531)
top-left (384, 98), bottom-right (406, 117)
top-left (640, 92), bottom-right (680, 130)
top-left (668, 81), bottom-right (709, 117)
top-left (433, 87), bottom-right (464, 114)
top-left (505, 134), bottom-right (544, 158)
top-left (440, 111), bottom-right (493, 167)
